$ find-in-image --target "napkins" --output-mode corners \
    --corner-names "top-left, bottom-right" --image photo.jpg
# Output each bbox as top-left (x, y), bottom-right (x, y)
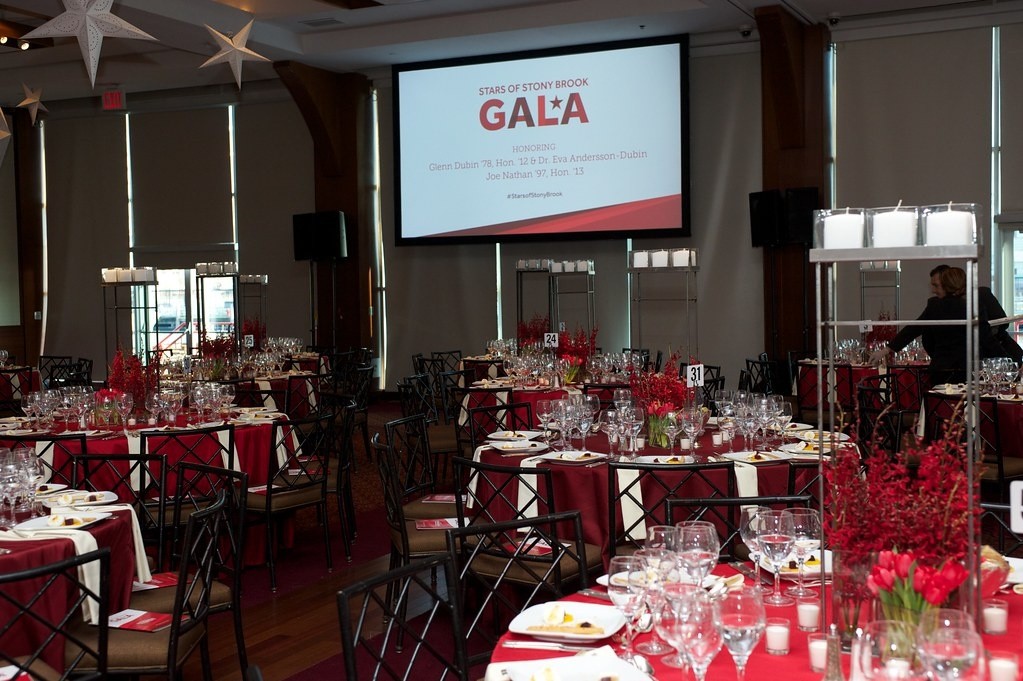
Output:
top-left (85, 503), bottom-right (152, 584)
top-left (562, 387), bottom-right (582, 395)
top-left (35, 441), bottom-right (54, 485)
top-left (9, 372), bottom-right (22, 400)
top-left (254, 377), bottom-right (276, 409)
top-left (320, 357), bottom-right (326, 374)
top-left (616, 468), bottom-right (647, 541)
top-left (517, 456), bottom-right (544, 533)
top-left (792, 359), bottom-right (811, 395)
top-left (878, 365), bottom-right (892, 403)
top-left (466, 445), bottom-right (494, 509)
top-left (707, 455), bottom-right (758, 529)
top-left (204, 422), bottom-right (243, 483)
top-left (484, 645), bottom-right (654, 681)
top-left (496, 391), bottom-right (509, 431)
top-left (276, 425), bottom-right (289, 469)
top-left (917, 397), bottom-right (925, 436)
top-left (488, 363), bottom-right (497, 380)
top-left (458, 394), bottom-right (470, 426)
top-left (45, 529), bottom-right (100, 625)
top-left (278, 413), bottom-right (303, 457)
top-left (302, 373), bottom-right (318, 413)
top-left (827, 367), bottom-right (838, 402)
top-left (964, 400), bottom-right (977, 450)
top-left (125, 429), bottom-right (150, 491)
top-left (458, 361), bottom-right (465, 387)
top-left (841, 442), bottom-right (866, 482)
top-left (292, 363), bottom-right (300, 371)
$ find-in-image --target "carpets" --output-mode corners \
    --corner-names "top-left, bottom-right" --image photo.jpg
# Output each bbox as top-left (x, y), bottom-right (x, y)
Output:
top-left (207, 403), bottom-right (456, 622)
top-left (284, 600), bottom-right (498, 681)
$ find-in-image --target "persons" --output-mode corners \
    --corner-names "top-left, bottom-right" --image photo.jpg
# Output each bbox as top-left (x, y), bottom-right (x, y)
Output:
top-left (867, 264), bottom-right (1023, 383)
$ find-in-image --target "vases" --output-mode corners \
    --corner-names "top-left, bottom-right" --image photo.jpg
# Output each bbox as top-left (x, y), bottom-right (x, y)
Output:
top-left (649, 418), bottom-right (675, 448)
top-left (835, 547), bottom-right (970, 657)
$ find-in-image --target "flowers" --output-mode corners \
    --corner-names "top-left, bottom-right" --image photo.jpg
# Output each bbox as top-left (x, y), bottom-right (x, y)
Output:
top-left (198, 316), bottom-right (266, 377)
top-left (94, 389), bottom-right (125, 424)
top-left (516, 312), bottom-right (551, 348)
top-left (866, 552), bottom-right (970, 651)
top-left (869, 340), bottom-right (885, 351)
top-left (866, 301), bottom-right (898, 363)
top-left (107, 344), bottom-right (163, 410)
top-left (648, 401), bottom-right (679, 447)
top-left (626, 342), bottom-right (705, 418)
top-left (556, 322), bottom-right (599, 383)
top-left (820, 384), bottom-right (988, 607)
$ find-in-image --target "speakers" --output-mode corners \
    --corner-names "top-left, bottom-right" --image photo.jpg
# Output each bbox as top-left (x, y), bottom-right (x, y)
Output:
top-left (748, 186), bottom-right (820, 248)
top-left (292, 211), bottom-right (348, 261)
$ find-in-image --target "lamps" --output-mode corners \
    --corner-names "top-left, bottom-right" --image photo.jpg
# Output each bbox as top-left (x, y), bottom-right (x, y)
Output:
top-left (18, 40), bottom-right (29, 50)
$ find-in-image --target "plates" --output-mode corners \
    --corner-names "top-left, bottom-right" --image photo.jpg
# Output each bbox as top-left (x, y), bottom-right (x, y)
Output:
top-left (256, 352), bottom-right (319, 380)
top-left (14, 481), bottom-right (119, 530)
top-left (537, 422), bottom-right (559, 430)
top-left (748, 550), bottom-right (832, 580)
top-left (508, 601), bottom-right (627, 644)
top-left (138, 403), bottom-right (278, 432)
top-left (484, 644), bottom-right (655, 681)
top-left (597, 570), bottom-right (721, 597)
top-left (1001, 556), bottom-right (1023, 584)
top-left (486, 415), bottom-right (849, 466)
top-left (0, 410), bottom-right (113, 436)
top-left (932, 380), bottom-right (1023, 402)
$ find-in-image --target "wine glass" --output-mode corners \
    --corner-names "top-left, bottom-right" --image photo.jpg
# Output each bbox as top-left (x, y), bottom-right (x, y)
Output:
top-left (253, 336), bottom-right (303, 375)
top-left (974, 357), bottom-right (1019, 396)
top-left (607, 506), bottom-right (823, 681)
top-left (659, 390), bottom-right (793, 456)
top-left (535, 390), bottom-right (643, 461)
top-left (21, 380), bottom-right (235, 436)
top-left (0, 350), bottom-right (9, 367)
top-left (0, 447), bottom-right (45, 528)
top-left (487, 338), bottom-right (571, 391)
top-left (586, 352), bottom-right (645, 384)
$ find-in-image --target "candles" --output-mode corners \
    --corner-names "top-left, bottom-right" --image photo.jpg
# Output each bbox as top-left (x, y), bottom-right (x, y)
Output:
top-left (240, 275), bottom-right (268, 283)
top-left (652, 249), bottom-right (668, 267)
top-left (672, 248), bottom-right (696, 266)
top-left (105, 268), bottom-right (154, 282)
top-left (634, 250), bottom-right (649, 267)
top-left (197, 264), bottom-right (236, 274)
top-left (927, 201), bottom-right (976, 246)
top-left (551, 260), bottom-right (592, 272)
top-left (872, 200), bottom-right (918, 248)
top-left (823, 207), bottom-right (865, 249)
top-left (518, 259), bottom-right (550, 269)
top-left (862, 260), bottom-right (899, 269)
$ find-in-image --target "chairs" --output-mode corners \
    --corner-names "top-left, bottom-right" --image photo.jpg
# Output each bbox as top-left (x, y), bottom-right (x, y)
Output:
top-left (0, 345), bottom-right (373, 681)
top-left (336, 347), bottom-right (1022, 681)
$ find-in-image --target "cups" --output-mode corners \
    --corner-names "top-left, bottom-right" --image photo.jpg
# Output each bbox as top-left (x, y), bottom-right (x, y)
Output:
top-left (601, 376), bottom-right (607, 383)
top-left (1016, 383), bottom-right (1023, 394)
top-left (679, 436), bottom-right (691, 451)
top-left (712, 432), bottom-right (723, 447)
top-left (610, 375), bottom-right (617, 383)
top-left (764, 616), bottom-right (791, 656)
top-left (831, 338), bottom-right (925, 364)
top-left (987, 650), bottom-right (1019, 681)
top-left (981, 597), bottom-right (1008, 635)
top-left (795, 597), bottom-right (822, 633)
top-left (636, 435), bottom-right (646, 451)
top-left (807, 633), bottom-right (829, 674)
top-left (859, 607), bottom-right (986, 681)
top-left (719, 429), bottom-right (729, 443)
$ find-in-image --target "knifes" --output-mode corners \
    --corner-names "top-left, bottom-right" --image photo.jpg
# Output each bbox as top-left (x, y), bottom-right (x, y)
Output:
top-left (727, 562), bottom-right (774, 586)
top-left (502, 640), bottom-right (617, 652)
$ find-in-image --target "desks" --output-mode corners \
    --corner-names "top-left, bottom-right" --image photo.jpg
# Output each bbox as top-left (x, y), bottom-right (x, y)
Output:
top-left (457, 354), bottom-right (510, 390)
top-left (0, 489), bottom-right (152, 675)
top-left (929, 385), bottom-right (1023, 459)
top-left (183, 372), bottom-right (322, 420)
top-left (0, 407), bottom-right (304, 579)
top-left (465, 376), bottom-right (630, 443)
top-left (464, 418), bottom-right (870, 631)
top-left (793, 358), bottom-right (930, 410)
top-left (276, 353), bottom-right (333, 391)
top-left (0, 365), bottom-right (44, 411)
